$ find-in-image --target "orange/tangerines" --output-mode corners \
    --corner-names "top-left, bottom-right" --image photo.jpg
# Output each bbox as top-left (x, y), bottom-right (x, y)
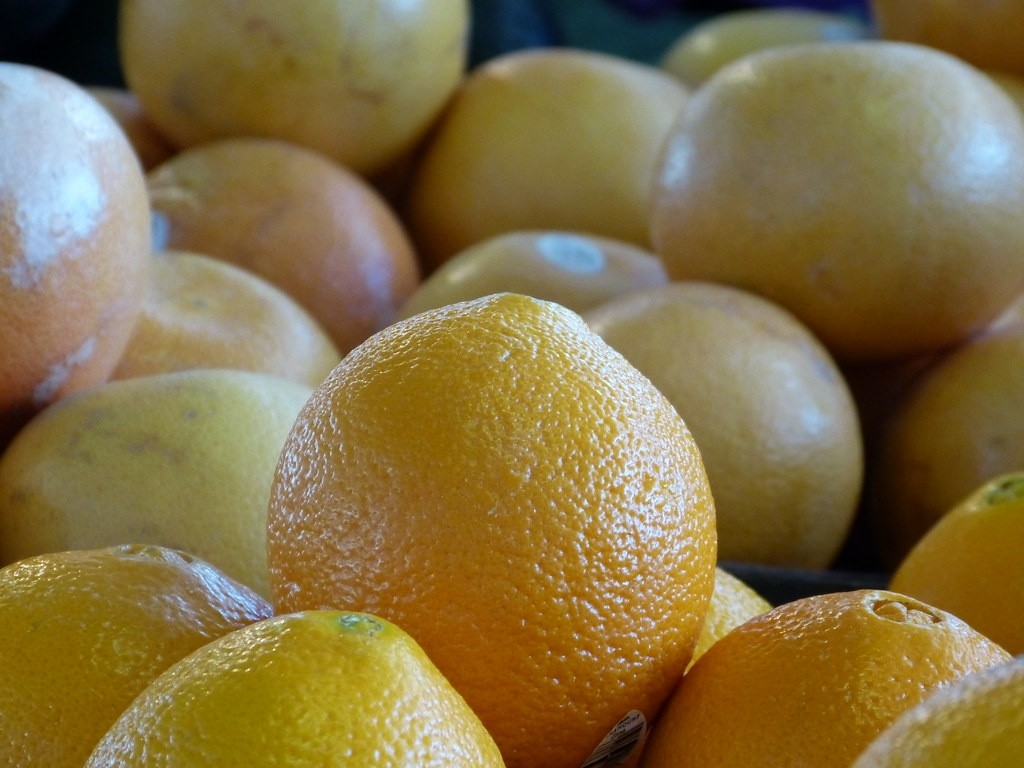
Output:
top-left (0, 0), bottom-right (1024, 577)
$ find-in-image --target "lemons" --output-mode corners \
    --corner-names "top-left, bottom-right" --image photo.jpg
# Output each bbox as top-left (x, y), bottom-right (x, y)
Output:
top-left (0, 290), bottom-right (1024, 768)
